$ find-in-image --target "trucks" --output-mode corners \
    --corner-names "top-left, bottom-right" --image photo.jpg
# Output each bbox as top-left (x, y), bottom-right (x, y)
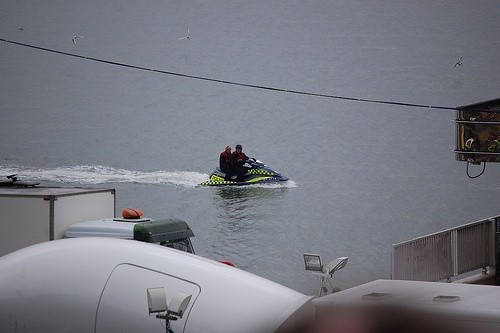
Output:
top-left (0, 174), bottom-right (196, 258)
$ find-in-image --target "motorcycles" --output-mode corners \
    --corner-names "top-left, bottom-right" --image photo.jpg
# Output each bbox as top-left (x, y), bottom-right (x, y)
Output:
top-left (197, 157), bottom-right (289, 187)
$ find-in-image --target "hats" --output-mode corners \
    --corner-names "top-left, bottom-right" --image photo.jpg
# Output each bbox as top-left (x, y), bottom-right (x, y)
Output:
top-left (225, 146), bottom-right (230, 151)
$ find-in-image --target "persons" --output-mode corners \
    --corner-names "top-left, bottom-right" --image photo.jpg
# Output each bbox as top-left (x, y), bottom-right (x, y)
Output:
top-left (220, 146), bottom-right (232, 180)
top-left (231, 145), bottom-right (256, 182)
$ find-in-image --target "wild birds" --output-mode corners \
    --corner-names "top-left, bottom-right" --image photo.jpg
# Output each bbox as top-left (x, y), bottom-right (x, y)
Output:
top-left (176, 25), bottom-right (192, 41)
top-left (452, 54), bottom-right (464, 68)
top-left (71, 34), bottom-right (86, 45)
top-left (16, 24), bottom-right (25, 31)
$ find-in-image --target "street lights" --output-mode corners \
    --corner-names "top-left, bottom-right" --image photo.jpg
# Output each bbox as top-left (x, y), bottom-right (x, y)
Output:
top-left (146, 285), bottom-right (193, 333)
top-left (302, 252), bottom-right (350, 298)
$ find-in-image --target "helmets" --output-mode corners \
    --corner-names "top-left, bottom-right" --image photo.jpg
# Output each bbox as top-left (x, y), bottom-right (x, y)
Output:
top-left (236, 145), bottom-right (242, 149)
